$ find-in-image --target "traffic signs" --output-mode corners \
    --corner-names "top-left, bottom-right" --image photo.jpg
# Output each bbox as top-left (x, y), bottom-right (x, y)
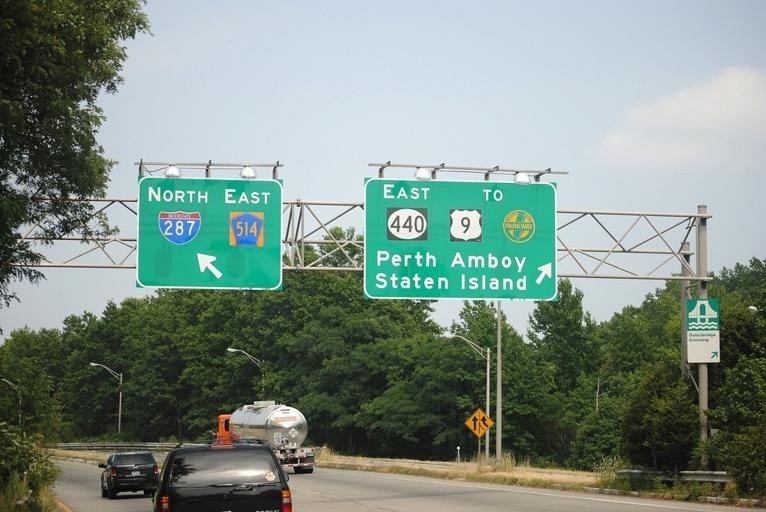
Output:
top-left (135, 175), bottom-right (281, 290)
top-left (362, 177), bottom-right (558, 303)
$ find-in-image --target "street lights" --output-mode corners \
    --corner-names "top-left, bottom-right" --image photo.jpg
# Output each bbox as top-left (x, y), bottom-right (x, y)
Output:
top-left (89, 362), bottom-right (122, 432)
top-left (226, 347), bottom-right (266, 401)
top-left (0, 378), bottom-right (21, 426)
top-left (745, 304), bottom-right (766, 315)
top-left (444, 329), bottom-right (491, 460)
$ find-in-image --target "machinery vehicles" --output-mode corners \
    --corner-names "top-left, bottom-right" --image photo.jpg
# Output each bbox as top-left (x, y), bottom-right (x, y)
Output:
top-left (204, 398), bottom-right (314, 474)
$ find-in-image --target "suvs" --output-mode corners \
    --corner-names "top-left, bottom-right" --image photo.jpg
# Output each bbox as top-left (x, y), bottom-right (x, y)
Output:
top-left (151, 437), bottom-right (295, 512)
top-left (96, 449), bottom-right (159, 501)
